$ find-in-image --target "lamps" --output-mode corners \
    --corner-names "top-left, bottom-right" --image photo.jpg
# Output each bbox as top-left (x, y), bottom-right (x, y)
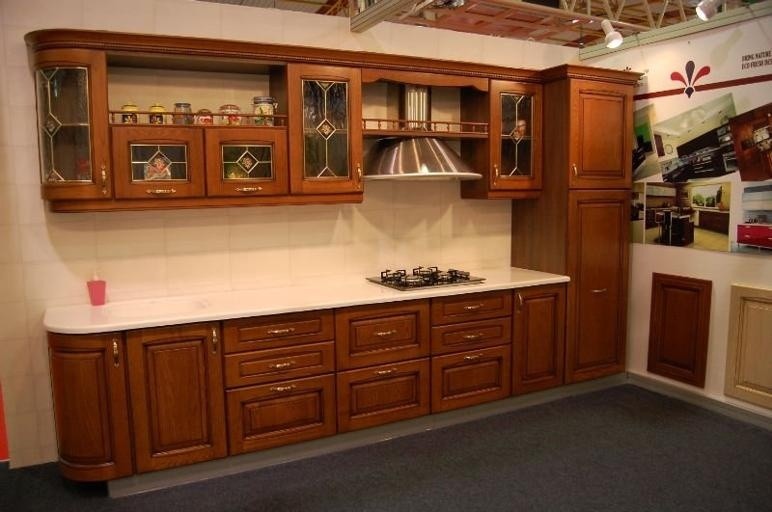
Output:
top-left (696, 1), bottom-right (725, 24)
top-left (599, 18), bottom-right (624, 49)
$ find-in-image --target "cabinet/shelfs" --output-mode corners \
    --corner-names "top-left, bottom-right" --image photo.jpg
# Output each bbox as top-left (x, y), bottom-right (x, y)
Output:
top-left (431, 292), bottom-right (512, 358)
top-left (461, 79), bottom-right (544, 199)
top-left (222, 308), bottom-right (336, 390)
top-left (431, 344), bottom-right (512, 413)
top-left (336, 299), bottom-right (429, 371)
top-left (269, 64), bottom-right (363, 206)
top-left (336, 358), bottom-right (431, 434)
top-left (45, 322), bottom-right (228, 500)
top-left (34, 48), bottom-right (112, 214)
top-left (513, 189), bottom-right (631, 410)
top-left (110, 124), bottom-right (288, 211)
top-left (226, 373), bottom-right (338, 458)
top-left (544, 78), bottom-right (633, 190)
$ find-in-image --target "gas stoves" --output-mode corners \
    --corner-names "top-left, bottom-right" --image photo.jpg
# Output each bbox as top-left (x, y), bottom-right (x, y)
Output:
top-left (366, 264), bottom-right (487, 292)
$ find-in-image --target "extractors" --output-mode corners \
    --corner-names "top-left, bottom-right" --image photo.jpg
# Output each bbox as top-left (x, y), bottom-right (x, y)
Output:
top-left (365, 84), bottom-right (486, 181)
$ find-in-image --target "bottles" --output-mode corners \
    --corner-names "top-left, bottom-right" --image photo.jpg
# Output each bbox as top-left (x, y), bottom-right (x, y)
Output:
top-left (123, 96), bottom-right (278, 127)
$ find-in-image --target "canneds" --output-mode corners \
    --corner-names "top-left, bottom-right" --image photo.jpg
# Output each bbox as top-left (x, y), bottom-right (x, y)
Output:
top-left (174, 103), bottom-right (192, 123)
top-left (219, 104), bottom-right (242, 125)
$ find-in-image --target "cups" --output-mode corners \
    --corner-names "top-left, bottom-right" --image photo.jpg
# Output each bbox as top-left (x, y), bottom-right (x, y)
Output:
top-left (88, 280), bottom-right (105, 306)
